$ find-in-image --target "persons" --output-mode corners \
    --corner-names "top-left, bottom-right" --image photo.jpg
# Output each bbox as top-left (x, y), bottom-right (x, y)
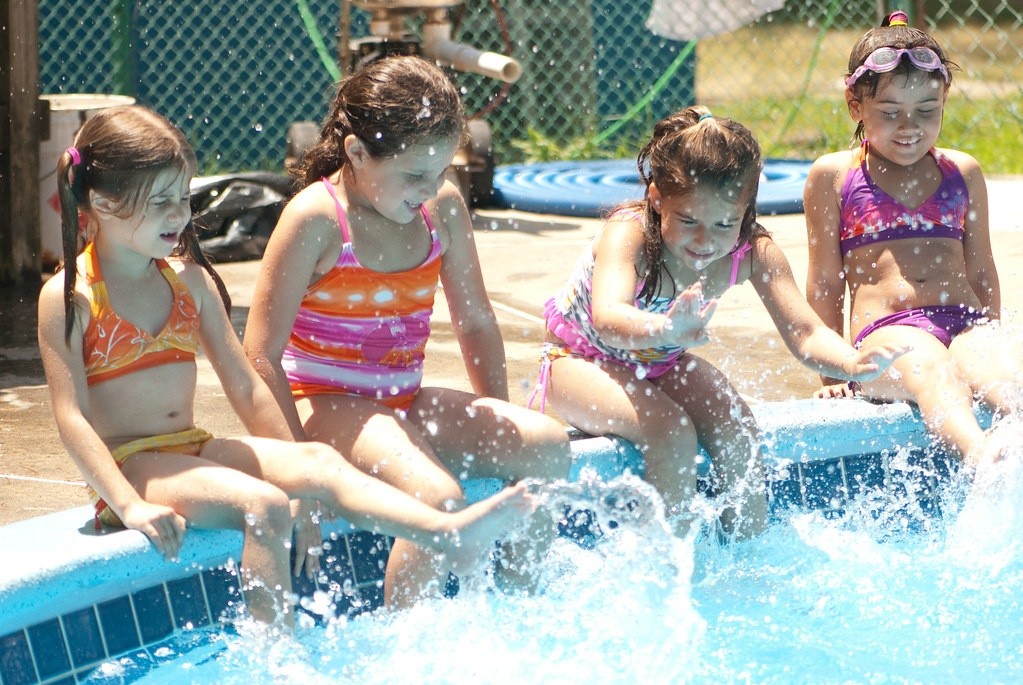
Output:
top-left (231, 51), bottom-right (574, 619)
top-left (800, 9), bottom-right (1016, 470)
top-left (37, 102), bottom-right (533, 653)
top-left (540, 100), bottom-right (916, 549)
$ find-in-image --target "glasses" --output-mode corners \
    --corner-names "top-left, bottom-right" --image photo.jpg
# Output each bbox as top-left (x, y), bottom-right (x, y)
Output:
top-left (845, 46), bottom-right (955, 95)
